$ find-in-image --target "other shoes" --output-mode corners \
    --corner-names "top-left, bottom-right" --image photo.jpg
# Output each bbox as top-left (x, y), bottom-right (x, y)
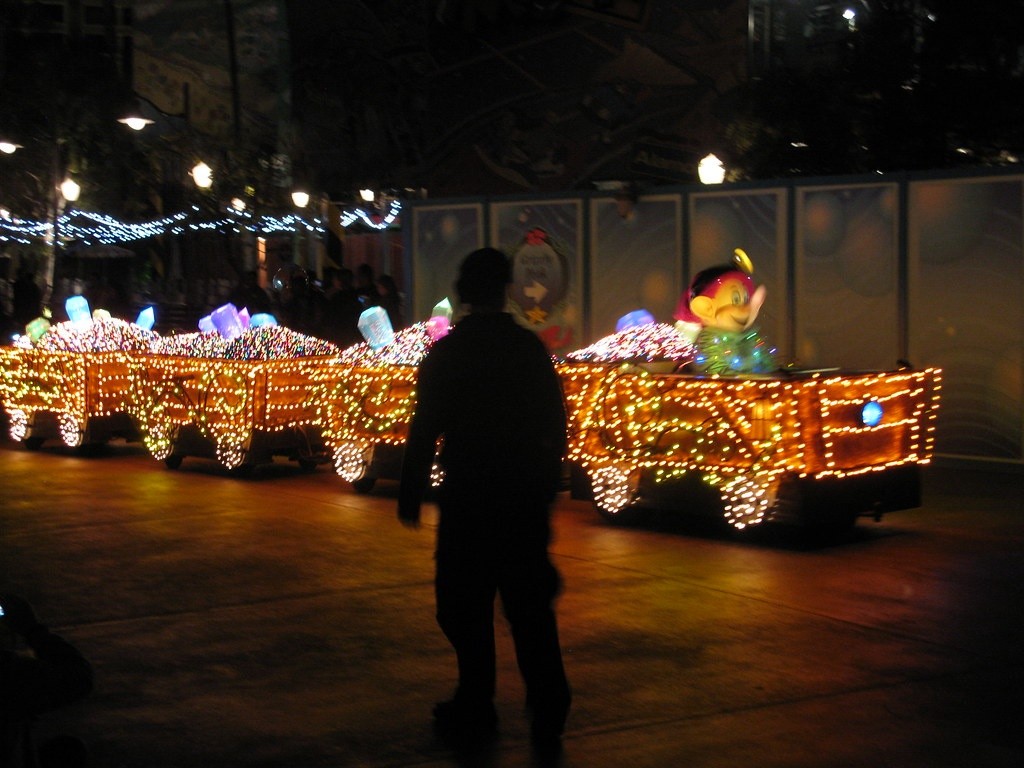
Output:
top-left (433, 689), bottom-right (496, 730)
top-left (529, 709), bottom-right (565, 738)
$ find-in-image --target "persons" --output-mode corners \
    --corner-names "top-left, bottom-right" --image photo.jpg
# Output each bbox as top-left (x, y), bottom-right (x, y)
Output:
top-left (12, 267), bottom-right (43, 322)
top-left (397, 245), bottom-right (569, 745)
top-left (242, 262), bottom-right (389, 347)
